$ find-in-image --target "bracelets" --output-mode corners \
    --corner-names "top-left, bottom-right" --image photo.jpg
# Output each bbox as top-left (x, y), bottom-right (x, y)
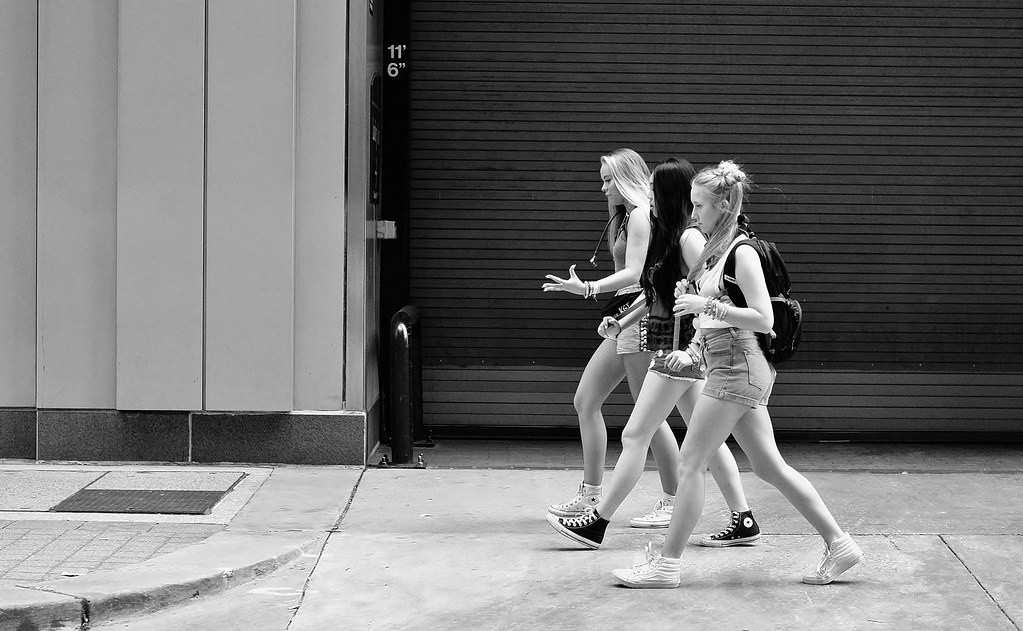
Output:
top-left (584, 280), bottom-right (601, 301)
top-left (705, 296), bottom-right (729, 322)
top-left (615, 327), bottom-right (622, 338)
top-left (686, 338), bottom-right (705, 364)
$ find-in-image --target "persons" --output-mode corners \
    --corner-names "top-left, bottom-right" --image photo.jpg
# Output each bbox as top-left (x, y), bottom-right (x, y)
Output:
top-left (610, 161), bottom-right (867, 589)
top-left (542, 148), bottom-right (680, 528)
top-left (545, 157), bottom-right (761, 550)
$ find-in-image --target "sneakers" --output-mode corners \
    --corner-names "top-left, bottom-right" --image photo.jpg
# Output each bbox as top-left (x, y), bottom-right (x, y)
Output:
top-left (611, 541), bottom-right (682, 588)
top-left (803, 531), bottom-right (864, 584)
top-left (701, 510), bottom-right (761, 546)
top-left (548, 481), bottom-right (603, 517)
top-left (545, 508), bottom-right (610, 549)
top-left (629, 496), bottom-right (676, 528)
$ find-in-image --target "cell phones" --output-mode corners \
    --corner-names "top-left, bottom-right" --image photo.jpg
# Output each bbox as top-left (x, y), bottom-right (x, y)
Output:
top-left (686, 284), bottom-right (696, 295)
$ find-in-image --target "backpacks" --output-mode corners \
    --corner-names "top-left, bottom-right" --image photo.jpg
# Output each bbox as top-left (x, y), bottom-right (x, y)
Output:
top-left (722, 238), bottom-right (803, 363)
